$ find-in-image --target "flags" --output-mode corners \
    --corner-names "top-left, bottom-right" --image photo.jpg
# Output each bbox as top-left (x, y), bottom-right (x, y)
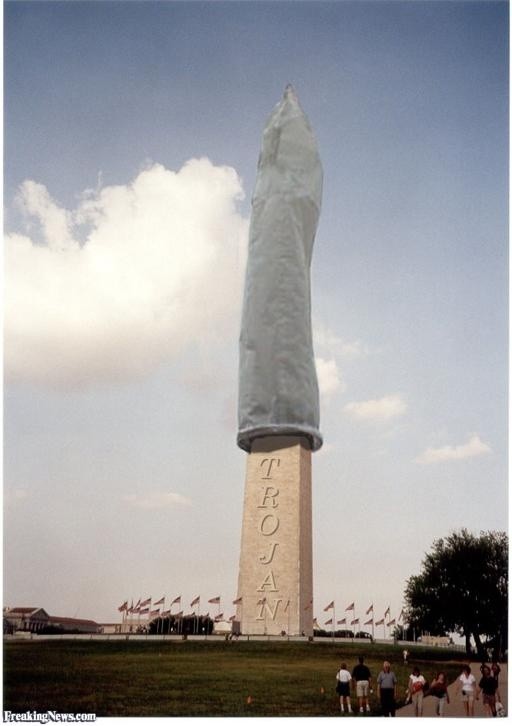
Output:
top-left (364, 604), bottom-right (395, 627)
top-left (325, 619), bottom-right (332, 625)
top-left (119, 596), bottom-right (242, 624)
top-left (345, 603), bottom-right (354, 610)
top-left (351, 619), bottom-right (359, 625)
top-left (324, 601), bottom-right (334, 611)
top-left (338, 619), bottom-right (346, 624)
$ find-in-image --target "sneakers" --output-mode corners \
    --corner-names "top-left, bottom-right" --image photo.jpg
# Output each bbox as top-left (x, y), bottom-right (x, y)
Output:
top-left (340, 705), bottom-right (373, 714)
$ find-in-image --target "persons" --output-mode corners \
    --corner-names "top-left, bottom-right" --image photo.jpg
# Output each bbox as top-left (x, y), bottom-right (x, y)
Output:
top-left (336, 663), bottom-right (353, 713)
top-left (351, 657), bottom-right (372, 713)
top-left (405, 667), bottom-right (427, 717)
top-left (479, 662), bottom-right (488, 678)
top-left (460, 665), bottom-right (478, 716)
top-left (490, 660), bottom-right (501, 680)
top-left (428, 667), bottom-right (450, 716)
top-left (377, 661), bottom-right (397, 717)
top-left (477, 666), bottom-right (500, 716)
